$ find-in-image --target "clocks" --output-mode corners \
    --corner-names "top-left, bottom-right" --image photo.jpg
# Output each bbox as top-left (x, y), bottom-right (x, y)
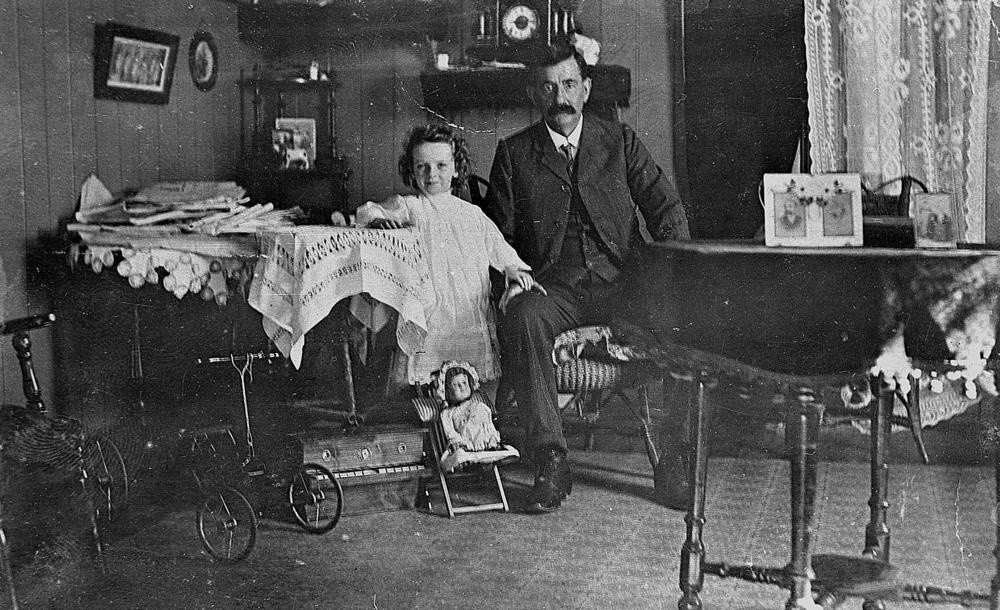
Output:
top-left (493, 0), bottom-right (570, 64)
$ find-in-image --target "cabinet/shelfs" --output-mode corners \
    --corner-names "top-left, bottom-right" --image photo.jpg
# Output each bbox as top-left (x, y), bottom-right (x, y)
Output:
top-left (243, 63), bottom-right (354, 221)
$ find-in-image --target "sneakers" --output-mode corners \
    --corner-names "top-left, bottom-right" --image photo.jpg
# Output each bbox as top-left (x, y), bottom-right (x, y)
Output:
top-left (527, 448), bottom-right (573, 512)
top-left (654, 447), bottom-right (692, 510)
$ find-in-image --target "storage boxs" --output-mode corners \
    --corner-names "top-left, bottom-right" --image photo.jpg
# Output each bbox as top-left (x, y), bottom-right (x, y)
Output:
top-left (284, 420), bottom-right (426, 520)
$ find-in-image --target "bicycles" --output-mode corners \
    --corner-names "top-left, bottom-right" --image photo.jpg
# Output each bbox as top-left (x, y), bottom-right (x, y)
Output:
top-left (124, 352), bottom-right (345, 564)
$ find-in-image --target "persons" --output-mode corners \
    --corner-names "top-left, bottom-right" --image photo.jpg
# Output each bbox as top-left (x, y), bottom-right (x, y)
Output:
top-left (779, 197), bottom-right (801, 230)
top-left (112, 44), bottom-right (210, 90)
top-left (484, 38), bottom-right (697, 511)
top-left (359, 121), bottom-right (535, 412)
top-left (918, 207), bottom-right (951, 242)
top-left (435, 361), bottom-right (504, 458)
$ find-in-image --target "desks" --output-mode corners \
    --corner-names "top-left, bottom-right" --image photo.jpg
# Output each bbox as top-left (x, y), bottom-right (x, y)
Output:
top-left (640, 237), bottom-right (1000, 609)
top-left (36, 227), bottom-right (436, 496)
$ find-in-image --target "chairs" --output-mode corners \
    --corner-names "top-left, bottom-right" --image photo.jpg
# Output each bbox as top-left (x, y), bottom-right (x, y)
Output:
top-left (411, 376), bottom-right (522, 517)
top-left (466, 173), bottom-right (662, 487)
top-left (0, 314), bottom-right (110, 610)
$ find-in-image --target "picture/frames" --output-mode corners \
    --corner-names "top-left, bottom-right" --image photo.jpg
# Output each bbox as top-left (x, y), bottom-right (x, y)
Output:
top-left (94, 22), bottom-right (179, 105)
top-left (763, 170), bottom-right (864, 249)
top-left (188, 31), bottom-right (220, 93)
